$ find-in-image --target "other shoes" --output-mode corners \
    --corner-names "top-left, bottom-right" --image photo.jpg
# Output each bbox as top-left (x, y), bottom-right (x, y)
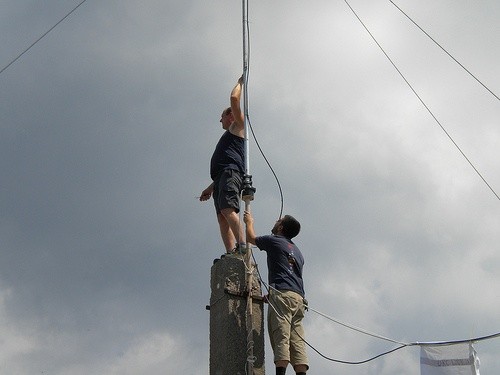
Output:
top-left (214, 259), bottom-right (220, 264)
top-left (221, 242), bottom-right (246, 260)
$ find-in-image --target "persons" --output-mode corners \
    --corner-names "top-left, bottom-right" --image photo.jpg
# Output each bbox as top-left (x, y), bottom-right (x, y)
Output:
top-left (243, 211), bottom-right (309, 375)
top-left (200, 74), bottom-right (246, 265)
